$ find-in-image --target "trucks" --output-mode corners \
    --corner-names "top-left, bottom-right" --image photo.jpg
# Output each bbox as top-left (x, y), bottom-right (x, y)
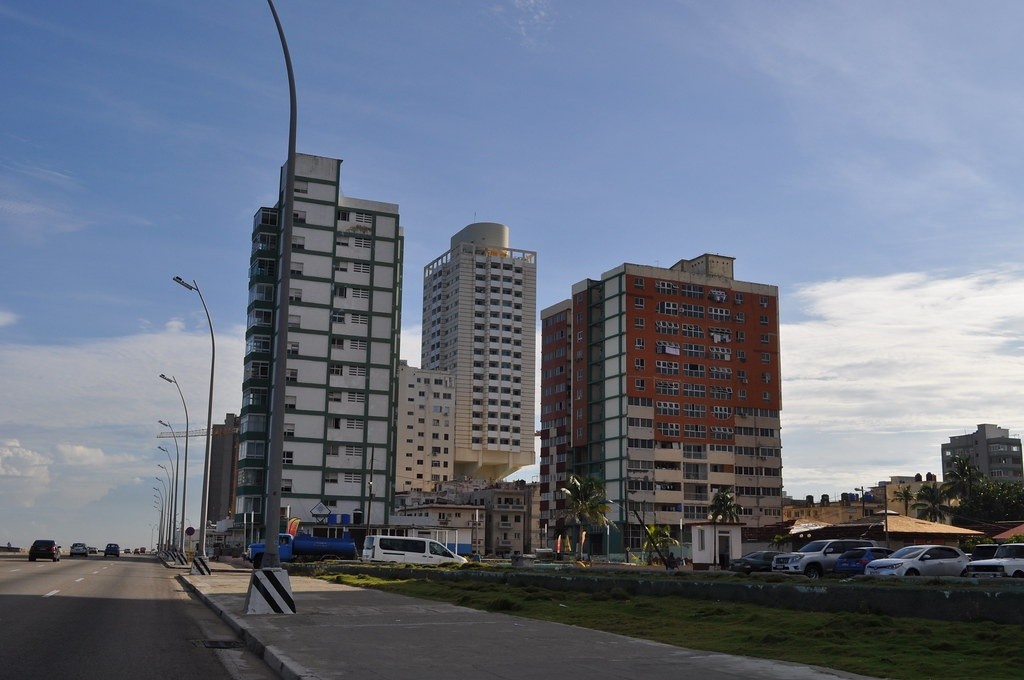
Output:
top-left (242, 533), bottom-right (357, 570)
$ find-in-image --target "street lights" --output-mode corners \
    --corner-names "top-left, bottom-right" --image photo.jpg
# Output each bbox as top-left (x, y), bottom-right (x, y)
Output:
top-left (172, 277), bottom-right (217, 556)
top-left (152, 370), bottom-right (189, 554)
top-left (149, 523), bottom-right (157, 550)
top-left (158, 445), bottom-right (174, 551)
top-left (855, 488), bottom-right (889, 548)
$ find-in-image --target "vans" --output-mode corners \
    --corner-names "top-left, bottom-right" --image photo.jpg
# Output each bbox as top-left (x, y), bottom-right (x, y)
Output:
top-left (772, 539), bottom-right (877, 579)
top-left (363, 534), bottom-right (468, 567)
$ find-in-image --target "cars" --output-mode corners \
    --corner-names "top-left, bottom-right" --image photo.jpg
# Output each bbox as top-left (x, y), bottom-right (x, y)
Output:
top-left (729, 551), bottom-right (784, 575)
top-left (87, 546), bottom-right (99, 554)
top-left (124, 548), bottom-right (132, 554)
top-left (104, 543), bottom-right (120, 557)
top-left (70, 542), bottom-right (88, 557)
top-left (134, 548), bottom-right (140, 554)
top-left (140, 547), bottom-right (146, 553)
top-left (832, 547), bottom-right (896, 577)
top-left (29, 539), bottom-right (61, 562)
top-left (864, 544), bottom-right (970, 577)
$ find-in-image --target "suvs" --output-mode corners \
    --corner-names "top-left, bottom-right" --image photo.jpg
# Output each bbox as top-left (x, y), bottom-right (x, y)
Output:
top-left (968, 543), bottom-right (1024, 578)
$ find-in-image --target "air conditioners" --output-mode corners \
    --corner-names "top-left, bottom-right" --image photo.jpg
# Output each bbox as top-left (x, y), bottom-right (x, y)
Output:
top-left (742, 413), bottom-right (748, 417)
top-left (743, 379), bottom-right (748, 383)
top-left (679, 308), bottom-right (684, 312)
top-left (433, 452), bottom-right (437, 456)
